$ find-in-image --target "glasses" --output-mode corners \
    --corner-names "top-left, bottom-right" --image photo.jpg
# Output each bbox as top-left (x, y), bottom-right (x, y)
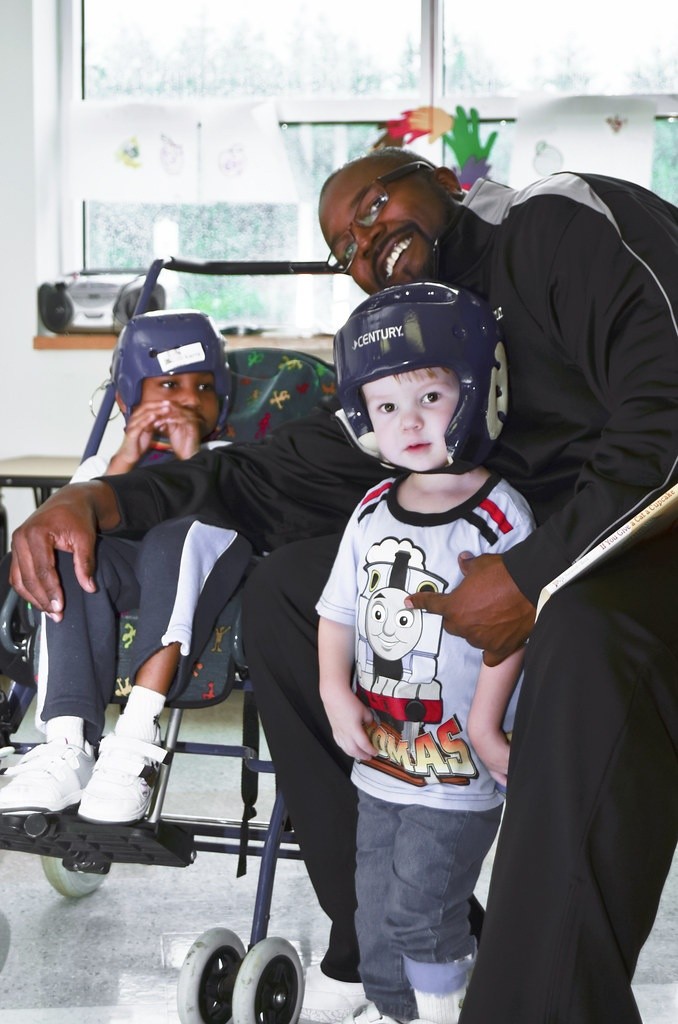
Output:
top-left (324, 161), bottom-right (435, 275)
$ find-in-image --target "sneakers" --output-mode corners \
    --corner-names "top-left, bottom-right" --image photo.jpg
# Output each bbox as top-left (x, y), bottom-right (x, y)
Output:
top-left (2, 744), bottom-right (95, 816)
top-left (78, 721), bottom-right (171, 825)
top-left (277, 967), bottom-right (369, 1023)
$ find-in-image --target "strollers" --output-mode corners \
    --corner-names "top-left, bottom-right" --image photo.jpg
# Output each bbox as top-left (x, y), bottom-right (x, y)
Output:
top-left (0, 254), bottom-right (363, 1023)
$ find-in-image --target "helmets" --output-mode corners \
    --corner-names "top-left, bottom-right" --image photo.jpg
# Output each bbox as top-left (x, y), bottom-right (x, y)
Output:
top-left (112, 309), bottom-right (232, 440)
top-left (334, 283), bottom-right (511, 470)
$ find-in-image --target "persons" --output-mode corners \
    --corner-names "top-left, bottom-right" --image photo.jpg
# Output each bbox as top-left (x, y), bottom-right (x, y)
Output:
top-left (8, 145), bottom-right (677, 1020)
top-left (307, 277), bottom-right (545, 1024)
top-left (0, 305), bottom-right (257, 830)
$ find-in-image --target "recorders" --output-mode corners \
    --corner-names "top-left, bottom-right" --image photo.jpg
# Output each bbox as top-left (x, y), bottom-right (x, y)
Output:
top-left (38, 269), bottom-right (167, 334)
